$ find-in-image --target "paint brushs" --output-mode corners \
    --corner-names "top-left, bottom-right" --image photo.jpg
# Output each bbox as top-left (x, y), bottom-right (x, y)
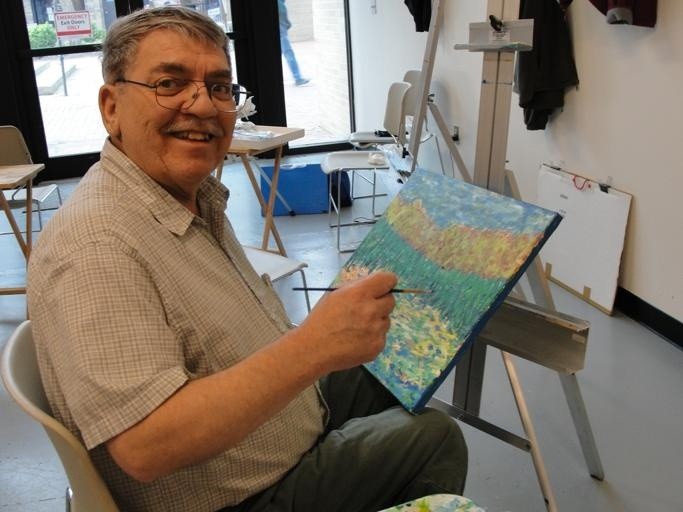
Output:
top-left (293, 288), bottom-right (434, 294)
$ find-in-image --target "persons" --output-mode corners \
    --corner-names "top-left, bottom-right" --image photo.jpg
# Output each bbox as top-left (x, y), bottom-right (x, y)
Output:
top-left (24, 5), bottom-right (469, 512)
top-left (278, 0), bottom-right (310, 87)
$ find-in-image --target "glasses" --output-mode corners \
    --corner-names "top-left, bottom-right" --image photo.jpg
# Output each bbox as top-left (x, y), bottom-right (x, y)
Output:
top-left (115, 76), bottom-right (252, 114)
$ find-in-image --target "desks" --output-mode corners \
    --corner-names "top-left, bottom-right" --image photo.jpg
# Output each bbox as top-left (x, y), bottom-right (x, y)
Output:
top-left (0, 164), bottom-right (45, 316)
top-left (215, 125), bottom-right (305, 258)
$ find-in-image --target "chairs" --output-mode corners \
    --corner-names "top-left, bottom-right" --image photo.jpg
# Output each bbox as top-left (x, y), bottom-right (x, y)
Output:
top-left (241, 245), bottom-right (310, 322)
top-left (350, 69), bottom-right (444, 217)
top-left (0, 320), bottom-right (130, 512)
top-left (321, 81), bottom-right (411, 253)
top-left (0, 126), bottom-right (62, 234)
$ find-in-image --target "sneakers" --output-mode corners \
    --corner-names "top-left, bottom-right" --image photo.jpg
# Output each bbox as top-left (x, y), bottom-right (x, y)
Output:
top-left (296, 78), bottom-right (309, 86)
